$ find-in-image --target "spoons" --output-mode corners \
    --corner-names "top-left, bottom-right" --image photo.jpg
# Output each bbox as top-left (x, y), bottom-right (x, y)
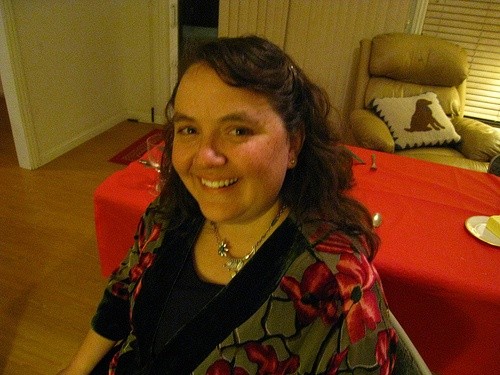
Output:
top-left (371, 213), bottom-right (382, 233)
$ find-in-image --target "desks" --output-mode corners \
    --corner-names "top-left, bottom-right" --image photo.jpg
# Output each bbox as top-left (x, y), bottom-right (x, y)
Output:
top-left (92, 138), bottom-right (500, 375)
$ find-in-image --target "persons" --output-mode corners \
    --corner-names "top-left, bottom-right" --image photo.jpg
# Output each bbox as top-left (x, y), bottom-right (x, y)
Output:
top-left (56, 36), bottom-right (399, 375)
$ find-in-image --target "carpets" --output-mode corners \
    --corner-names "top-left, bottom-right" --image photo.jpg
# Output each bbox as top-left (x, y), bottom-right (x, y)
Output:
top-left (108, 128), bottom-right (165, 165)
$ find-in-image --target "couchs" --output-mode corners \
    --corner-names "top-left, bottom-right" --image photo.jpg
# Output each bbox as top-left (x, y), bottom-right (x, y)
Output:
top-left (349, 33), bottom-right (500, 174)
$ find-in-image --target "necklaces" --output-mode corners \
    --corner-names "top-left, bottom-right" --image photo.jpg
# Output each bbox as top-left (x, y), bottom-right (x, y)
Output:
top-left (209, 196), bottom-right (290, 278)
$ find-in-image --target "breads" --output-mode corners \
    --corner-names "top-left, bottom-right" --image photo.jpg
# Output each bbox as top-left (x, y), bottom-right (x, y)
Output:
top-left (487, 215), bottom-right (500, 239)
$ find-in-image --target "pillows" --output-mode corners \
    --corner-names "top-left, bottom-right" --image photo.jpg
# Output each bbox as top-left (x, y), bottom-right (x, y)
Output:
top-left (372, 92), bottom-right (462, 152)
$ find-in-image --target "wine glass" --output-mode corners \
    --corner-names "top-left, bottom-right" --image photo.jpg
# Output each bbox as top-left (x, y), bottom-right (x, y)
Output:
top-left (147, 135), bottom-right (168, 196)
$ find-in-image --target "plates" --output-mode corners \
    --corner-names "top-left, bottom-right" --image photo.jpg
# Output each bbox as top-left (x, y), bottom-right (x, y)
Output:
top-left (465, 215), bottom-right (500, 246)
